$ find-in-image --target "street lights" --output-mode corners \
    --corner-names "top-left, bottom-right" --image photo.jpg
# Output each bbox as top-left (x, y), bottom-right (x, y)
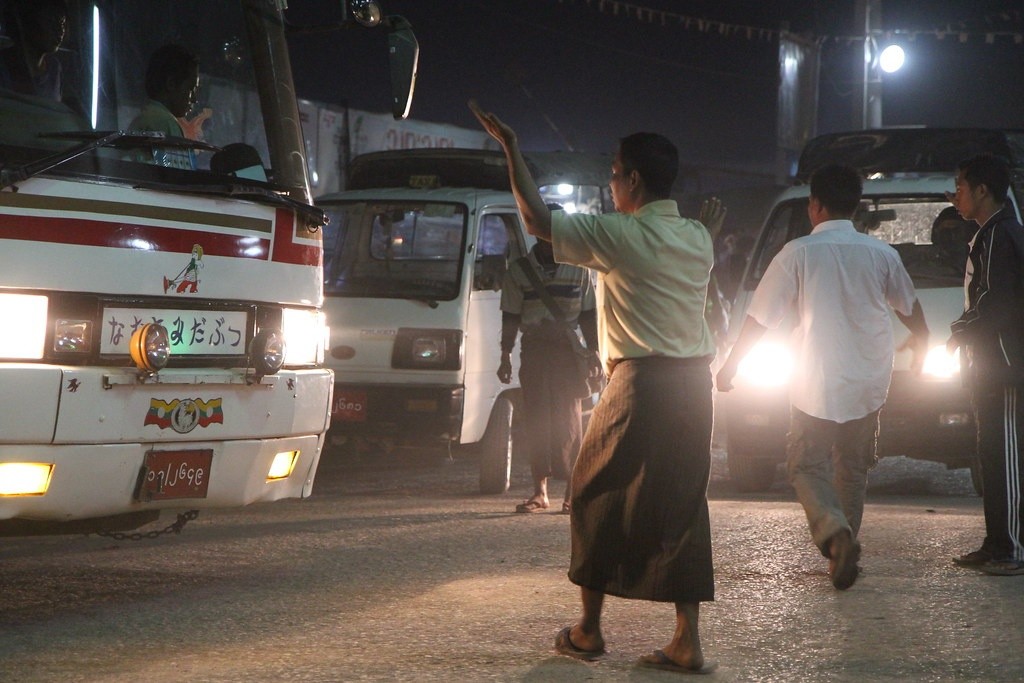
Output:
top-left (855, 39), bottom-right (905, 128)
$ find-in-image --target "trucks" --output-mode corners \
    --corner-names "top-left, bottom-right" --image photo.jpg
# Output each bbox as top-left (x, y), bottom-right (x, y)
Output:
top-left (311, 146), bottom-right (634, 492)
top-left (723, 125), bottom-right (1023, 492)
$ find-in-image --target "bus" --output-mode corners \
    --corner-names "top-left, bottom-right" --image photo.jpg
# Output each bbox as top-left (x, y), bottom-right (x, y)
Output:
top-left (0, 0), bottom-right (422, 544)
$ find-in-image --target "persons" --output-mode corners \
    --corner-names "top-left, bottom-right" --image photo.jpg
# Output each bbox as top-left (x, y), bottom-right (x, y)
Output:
top-left (0, 0), bottom-right (66, 113)
top-left (923, 206), bottom-right (981, 265)
top-left (127, 44), bottom-right (203, 167)
top-left (715, 164), bottom-right (931, 590)
top-left (946, 153), bottom-right (1024, 576)
top-left (496, 202), bottom-right (604, 514)
top-left (468, 100), bottom-right (720, 675)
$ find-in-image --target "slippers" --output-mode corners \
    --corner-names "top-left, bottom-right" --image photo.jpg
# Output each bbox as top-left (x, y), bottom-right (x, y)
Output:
top-left (555, 626), bottom-right (605, 661)
top-left (982, 562), bottom-right (1024, 575)
top-left (952, 550), bottom-right (991, 565)
top-left (516, 497), bottom-right (551, 514)
top-left (638, 650), bottom-right (718, 674)
top-left (562, 502), bottom-right (571, 514)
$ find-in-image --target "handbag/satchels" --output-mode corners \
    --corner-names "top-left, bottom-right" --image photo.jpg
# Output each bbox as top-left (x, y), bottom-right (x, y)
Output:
top-left (566, 332), bottom-right (608, 398)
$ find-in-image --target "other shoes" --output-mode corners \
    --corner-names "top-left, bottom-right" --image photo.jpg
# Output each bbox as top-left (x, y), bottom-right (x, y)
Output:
top-left (829, 532), bottom-right (861, 591)
